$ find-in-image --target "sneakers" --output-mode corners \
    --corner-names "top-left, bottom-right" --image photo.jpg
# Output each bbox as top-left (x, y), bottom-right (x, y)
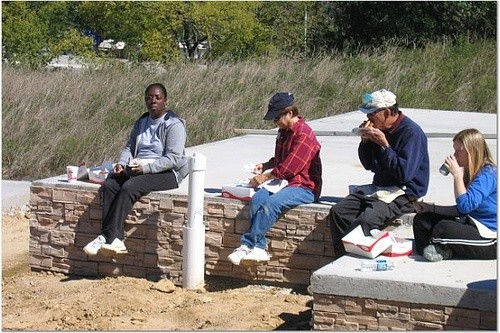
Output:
top-left (83, 235), bottom-right (106, 256)
top-left (226, 245), bottom-right (251, 266)
top-left (240, 248), bottom-right (270, 266)
top-left (100, 242), bottom-right (129, 257)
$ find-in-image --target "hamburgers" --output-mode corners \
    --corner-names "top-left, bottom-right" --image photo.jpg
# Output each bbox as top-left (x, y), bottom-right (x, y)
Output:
top-left (363, 120), bottom-right (374, 129)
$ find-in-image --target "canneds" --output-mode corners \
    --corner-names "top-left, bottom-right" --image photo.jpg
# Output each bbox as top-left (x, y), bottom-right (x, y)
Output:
top-left (439, 162), bottom-right (450, 175)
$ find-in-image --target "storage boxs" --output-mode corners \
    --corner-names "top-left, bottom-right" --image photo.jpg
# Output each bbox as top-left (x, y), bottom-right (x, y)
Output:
top-left (222, 183), bottom-right (257, 201)
top-left (342, 225), bottom-right (413, 259)
top-left (77, 165), bottom-right (109, 184)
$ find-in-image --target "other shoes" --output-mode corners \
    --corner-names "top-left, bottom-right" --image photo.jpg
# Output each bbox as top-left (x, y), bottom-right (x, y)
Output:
top-left (422, 245), bottom-right (452, 262)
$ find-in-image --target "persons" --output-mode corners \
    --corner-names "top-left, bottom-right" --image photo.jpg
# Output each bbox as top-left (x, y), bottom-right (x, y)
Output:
top-left (331, 89), bottom-right (430, 258)
top-left (410, 129), bottom-right (498, 263)
top-left (227, 93), bottom-right (321, 265)
top-left (82, 83), bottom-right (189, 256)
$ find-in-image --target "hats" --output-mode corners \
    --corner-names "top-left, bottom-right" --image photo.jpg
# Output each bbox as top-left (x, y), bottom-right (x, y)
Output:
top-left (262, 92), bottom-right (294, 120)
top-left (358, 89), bottom-right (397, 114)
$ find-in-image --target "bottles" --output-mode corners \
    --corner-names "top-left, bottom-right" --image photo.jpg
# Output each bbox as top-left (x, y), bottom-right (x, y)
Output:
top-left (440, 165), bottom-right (449, 176)
top-left (361, 259), bottom-right (397, 272)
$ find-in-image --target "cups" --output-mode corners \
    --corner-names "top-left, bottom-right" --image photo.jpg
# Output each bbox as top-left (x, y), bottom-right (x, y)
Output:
top-left (66, 166), bottom-right (78, 183)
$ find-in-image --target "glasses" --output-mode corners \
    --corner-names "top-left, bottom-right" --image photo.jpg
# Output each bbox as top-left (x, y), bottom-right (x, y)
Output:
top-left (273, 112), bottom-right (288, 123)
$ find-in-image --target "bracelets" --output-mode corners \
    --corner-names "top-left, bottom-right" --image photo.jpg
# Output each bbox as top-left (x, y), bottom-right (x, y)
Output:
top-left (433, 204), bottom-right (436, 214)
